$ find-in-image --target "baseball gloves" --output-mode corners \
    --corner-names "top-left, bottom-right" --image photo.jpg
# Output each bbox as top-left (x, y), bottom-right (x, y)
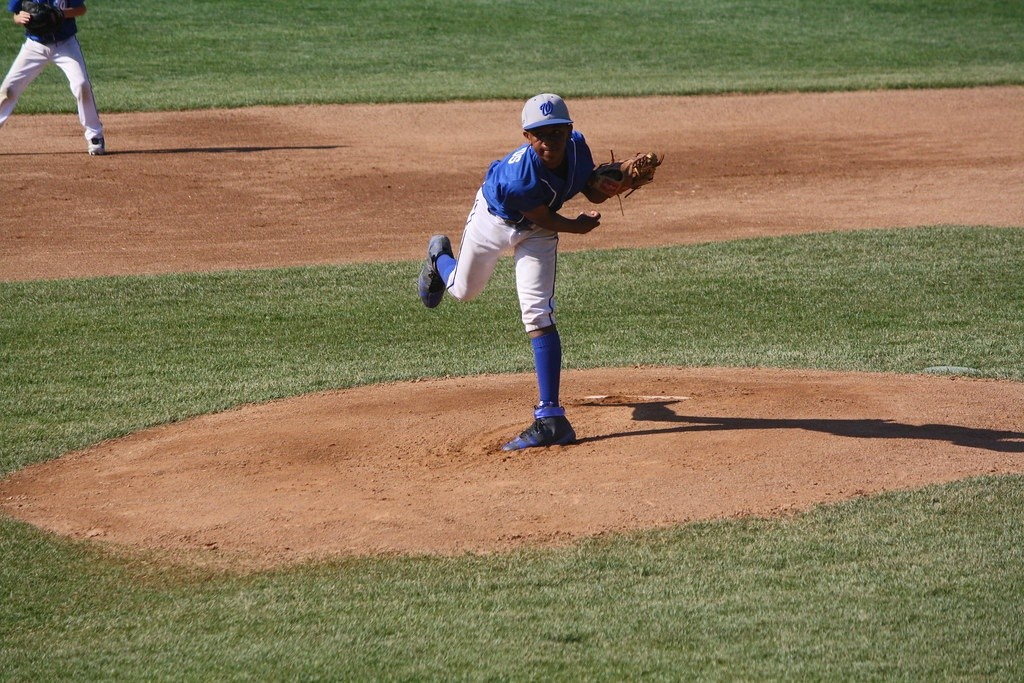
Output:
top-left (22, 0), bottom-right (65, 48)
top-left (592, 148), bottom-right (667, 217)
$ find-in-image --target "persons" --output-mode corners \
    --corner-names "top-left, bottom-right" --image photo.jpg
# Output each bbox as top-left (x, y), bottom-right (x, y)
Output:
top-left (0, 0), bottom-right (105, 156)
top-left (418, 93), bottom-right (665, 452)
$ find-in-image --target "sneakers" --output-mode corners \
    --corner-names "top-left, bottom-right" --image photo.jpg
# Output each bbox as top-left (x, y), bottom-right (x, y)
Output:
top-left (501, 405), bottom-right (577, 451)
top-left (87, 137), bottom-right (105, 156)
top-left (418, 235), bottom-right (454, 309)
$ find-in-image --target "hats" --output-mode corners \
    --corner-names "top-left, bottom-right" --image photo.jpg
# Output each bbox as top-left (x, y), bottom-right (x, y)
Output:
top-left (521, 93), bottom-right (574, 130)
